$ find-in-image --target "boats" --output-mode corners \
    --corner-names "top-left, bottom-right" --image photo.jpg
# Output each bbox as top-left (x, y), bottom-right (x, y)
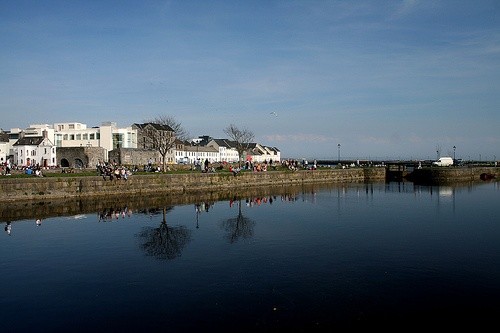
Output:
top-left (480, 173), bottom-right (497, 180)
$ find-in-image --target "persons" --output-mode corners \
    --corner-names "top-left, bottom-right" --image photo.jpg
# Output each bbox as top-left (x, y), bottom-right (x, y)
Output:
top-left (0, 155), bottom-right (424, 181)
top-left (455, 160), bottom-right (498, 167)
top-left (4, 187), bottom-right (318, 237)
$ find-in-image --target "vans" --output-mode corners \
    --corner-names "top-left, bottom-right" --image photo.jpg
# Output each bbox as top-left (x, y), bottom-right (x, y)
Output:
top-left (432, 156), bottom-right (454, 167)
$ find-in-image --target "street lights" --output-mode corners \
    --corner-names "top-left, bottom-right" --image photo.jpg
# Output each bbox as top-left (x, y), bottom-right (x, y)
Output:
top-left (453, 146), bottom-right (456, 167)
